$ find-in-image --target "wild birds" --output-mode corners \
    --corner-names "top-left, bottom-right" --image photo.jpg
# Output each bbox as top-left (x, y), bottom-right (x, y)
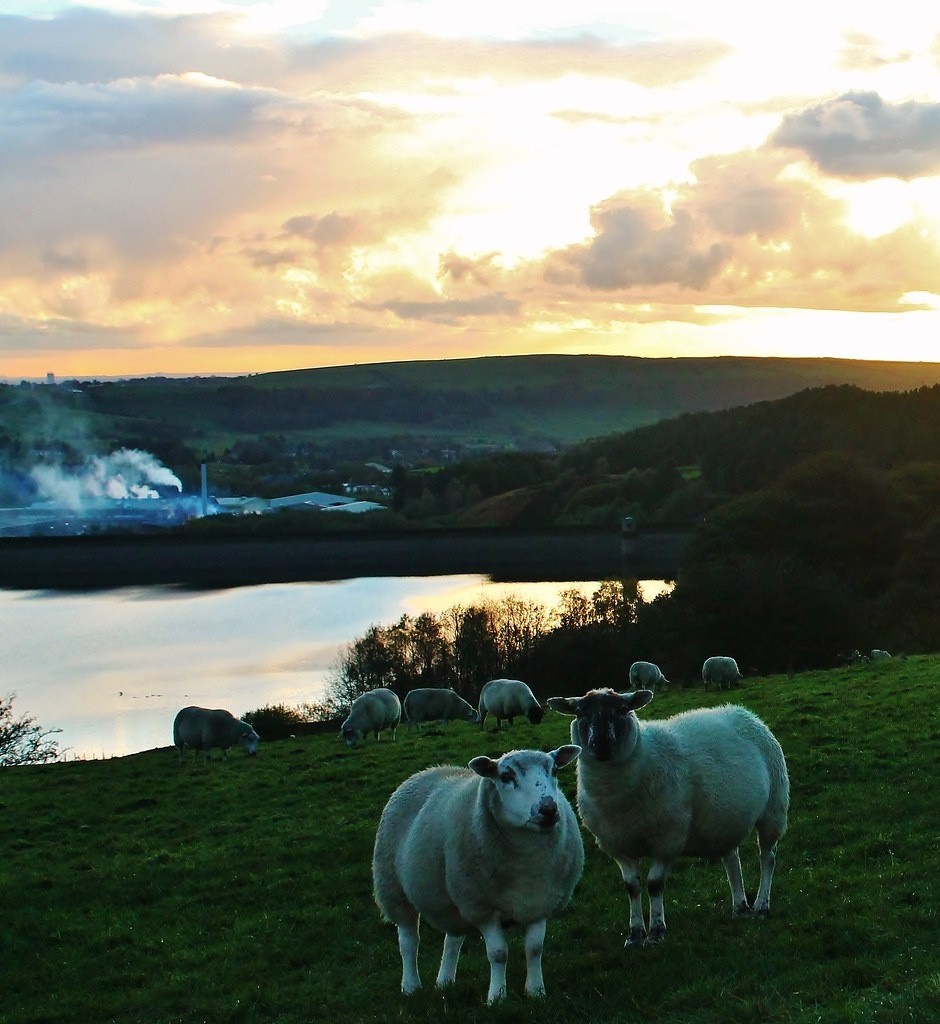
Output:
top-left (119, 691), bottom-right (191, 701)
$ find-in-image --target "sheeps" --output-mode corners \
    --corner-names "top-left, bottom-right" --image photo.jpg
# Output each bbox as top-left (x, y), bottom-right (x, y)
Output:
top-left (702, 655), bottom-right (745, 692)
top-left (629, 661), bottom-right (669, 696)
top-left (404, 688), bottom-right (482, 734)
top-left (478, 678), bottom-right (545, 731)
top-left (173, 706), bottom-right (262, 764)
top-left (370, 744), bottom-right (586, 1009)
top-left (546, 686), bottom-right (790, 949)
top-left (335, 688), bottom-right (402, 751)
top-left (871, 649), bottom-right (892, 659)
